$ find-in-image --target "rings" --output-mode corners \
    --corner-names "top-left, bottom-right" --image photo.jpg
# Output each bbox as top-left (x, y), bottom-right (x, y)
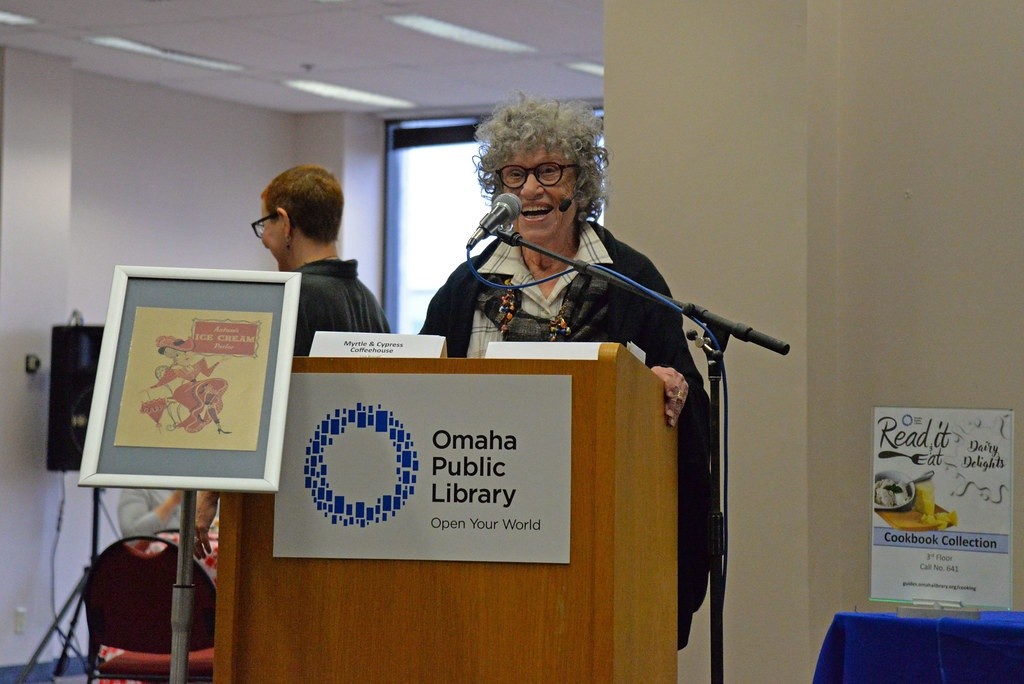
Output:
top-left (194, 537), bottom-right (200, 545)
top-left (678, 392), bottom-right (683, 401)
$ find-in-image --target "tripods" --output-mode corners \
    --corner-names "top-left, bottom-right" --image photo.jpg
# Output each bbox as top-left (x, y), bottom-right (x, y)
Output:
top-left (16, 486), bottom-right (105, 684)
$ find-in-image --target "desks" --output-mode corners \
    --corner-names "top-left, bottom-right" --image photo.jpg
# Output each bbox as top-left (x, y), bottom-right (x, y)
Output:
top-left (100, 533), bottom-right (219, 684)
top-left (812, 611), bottom-right (1024, 684)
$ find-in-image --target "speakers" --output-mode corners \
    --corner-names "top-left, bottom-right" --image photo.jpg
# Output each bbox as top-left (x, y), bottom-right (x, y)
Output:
top-left (46, 325), bottom-right (105, 471)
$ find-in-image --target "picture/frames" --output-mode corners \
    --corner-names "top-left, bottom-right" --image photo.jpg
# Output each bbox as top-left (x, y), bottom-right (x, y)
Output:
top-left (74, 264), bottom-right (304, 494)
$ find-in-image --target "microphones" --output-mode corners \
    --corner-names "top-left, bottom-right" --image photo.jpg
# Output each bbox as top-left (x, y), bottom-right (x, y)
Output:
top-left (466, 193), bottom-right (523, 250)
top-left (559, 193), bottom-right (580, 212)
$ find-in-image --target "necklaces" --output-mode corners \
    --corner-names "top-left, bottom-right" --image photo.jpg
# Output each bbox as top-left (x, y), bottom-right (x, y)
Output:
top-left (499, 279), bottom-right (571, 342)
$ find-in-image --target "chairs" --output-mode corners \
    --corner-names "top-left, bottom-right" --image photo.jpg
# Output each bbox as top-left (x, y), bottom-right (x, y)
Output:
top-left (81, 528), bottom-right (217, 684)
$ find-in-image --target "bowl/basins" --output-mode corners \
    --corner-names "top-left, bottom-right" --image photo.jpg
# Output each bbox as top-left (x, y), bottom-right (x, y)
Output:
top-left (874, 471), bottom-right (916, 510)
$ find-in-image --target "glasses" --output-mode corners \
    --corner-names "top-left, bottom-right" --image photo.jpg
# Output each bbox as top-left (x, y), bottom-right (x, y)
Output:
top-left (252, 213), bottom-right (295, 238)
top-left (495, 162), bottom-right (577, 189)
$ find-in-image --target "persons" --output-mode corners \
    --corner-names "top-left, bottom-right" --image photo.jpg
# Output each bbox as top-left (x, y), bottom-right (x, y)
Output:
top-left (117, 489), bottom-right (181, 545)
top-left (193, 165), bottom-right (391, 561)
top-left (421, 100), bottom-right (709, 651)
